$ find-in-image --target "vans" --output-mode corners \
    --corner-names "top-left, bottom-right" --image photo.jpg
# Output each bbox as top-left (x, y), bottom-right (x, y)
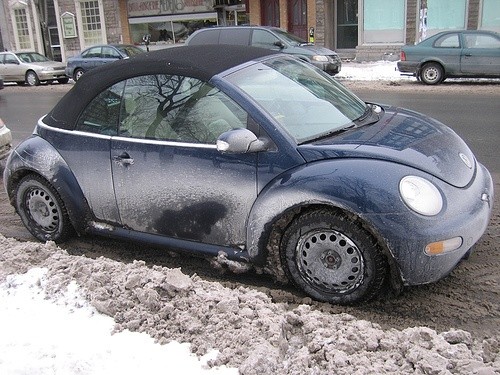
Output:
top-left (0, 49), bottom-right (69, 86)
top-left (185, 26), bottom-right (343, 76)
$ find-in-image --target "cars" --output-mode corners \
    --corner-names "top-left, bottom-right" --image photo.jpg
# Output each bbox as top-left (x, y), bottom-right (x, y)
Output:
top-left (397, 29), bottom-right (499, 87)
top-left (64, 43), bottom-right (148, 85)
top-left (2, 44), bottom-right (495, 309)
top-left (0, 117), bottom-right (13, 161)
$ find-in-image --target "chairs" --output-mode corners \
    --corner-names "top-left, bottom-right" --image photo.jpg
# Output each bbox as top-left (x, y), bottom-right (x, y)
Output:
top-left (121, 86), bottom-right (155, 138)
top-left (181, 79), bottom-right (244, 144)
